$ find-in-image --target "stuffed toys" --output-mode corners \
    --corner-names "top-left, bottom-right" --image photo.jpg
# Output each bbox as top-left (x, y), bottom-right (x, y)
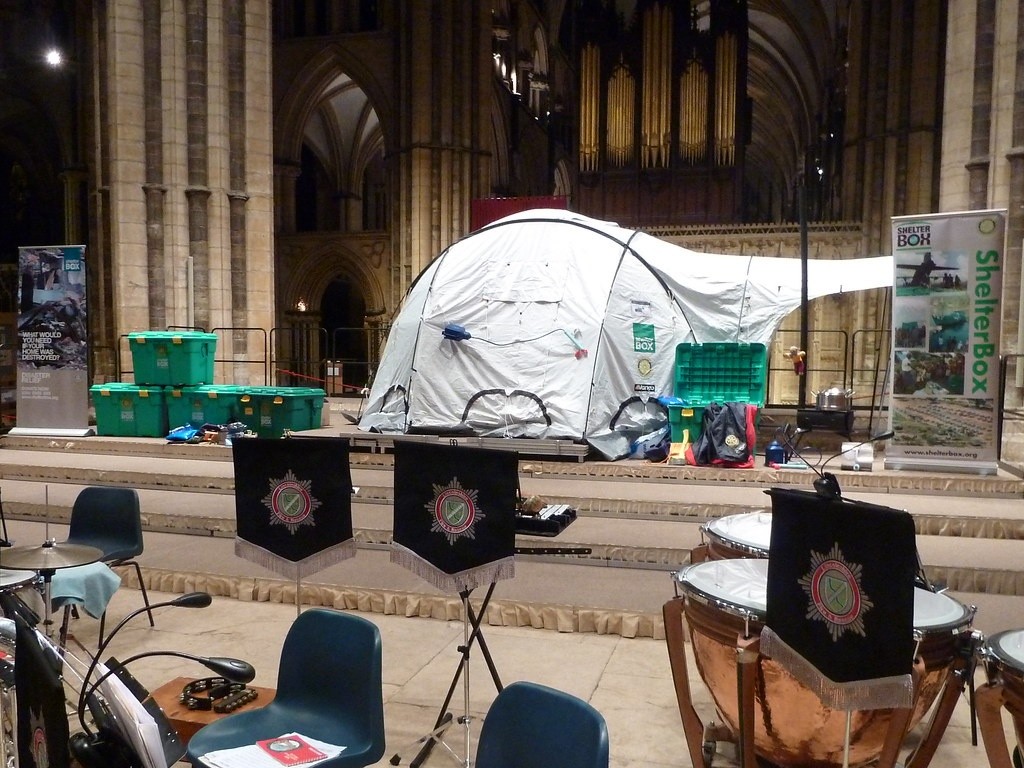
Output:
top-left (783, 346), bottom-right (805, 376)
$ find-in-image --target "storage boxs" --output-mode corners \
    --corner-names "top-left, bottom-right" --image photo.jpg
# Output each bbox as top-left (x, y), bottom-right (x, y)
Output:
top-left (668, 342), bottom-right (766, 461)
top-left (93, 331), bottom-right (329, 438)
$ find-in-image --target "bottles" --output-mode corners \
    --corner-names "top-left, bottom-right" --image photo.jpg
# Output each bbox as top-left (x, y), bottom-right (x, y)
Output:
top-left (169, 425), bottom-right (185, 435)
top-left (185, 423), bottom-right (191, 430)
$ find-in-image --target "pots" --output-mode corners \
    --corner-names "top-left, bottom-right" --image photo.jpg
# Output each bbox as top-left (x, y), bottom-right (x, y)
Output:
top-left (810, 387), bottom-right (856, 411)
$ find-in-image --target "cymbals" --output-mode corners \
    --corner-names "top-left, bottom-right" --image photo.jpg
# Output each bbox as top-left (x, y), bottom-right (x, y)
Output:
top-left (0, 543), bottom-right (104, 570)
top-left (179, 677), bottom-right (246, 710)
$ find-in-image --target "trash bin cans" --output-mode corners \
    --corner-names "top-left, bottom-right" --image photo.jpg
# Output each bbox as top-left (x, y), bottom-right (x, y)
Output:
top-left (324, 359), bottom-right (344, 397)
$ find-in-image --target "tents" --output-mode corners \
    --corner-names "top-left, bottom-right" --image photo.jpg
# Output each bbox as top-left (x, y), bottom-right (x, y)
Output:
top-left (358, 207), bottom-right (894, 458)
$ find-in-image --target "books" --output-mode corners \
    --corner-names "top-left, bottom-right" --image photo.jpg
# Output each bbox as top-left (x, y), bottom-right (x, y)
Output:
top-left (256, 735), bottom-right (328, 766)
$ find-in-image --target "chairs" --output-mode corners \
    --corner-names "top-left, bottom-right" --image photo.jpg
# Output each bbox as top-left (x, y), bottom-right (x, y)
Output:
top-left (188, 609), bottom-right (607, 768)
top-left (56, 486), bottom-right (152, 644)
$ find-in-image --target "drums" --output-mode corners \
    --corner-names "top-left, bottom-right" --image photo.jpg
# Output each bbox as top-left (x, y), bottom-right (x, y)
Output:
top-left (0, 568), bottom-right (46, 629)
top-left (685, 509), bottom-right (773, 562)
top-left (974, 628), bottom-right (1024, 768)
top-left (664, 558), bottom-right (983, 768)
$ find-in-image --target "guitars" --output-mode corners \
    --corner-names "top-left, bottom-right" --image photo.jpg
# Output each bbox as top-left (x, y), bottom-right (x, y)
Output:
top-left (0, 590), bottom-right (187, 768)
